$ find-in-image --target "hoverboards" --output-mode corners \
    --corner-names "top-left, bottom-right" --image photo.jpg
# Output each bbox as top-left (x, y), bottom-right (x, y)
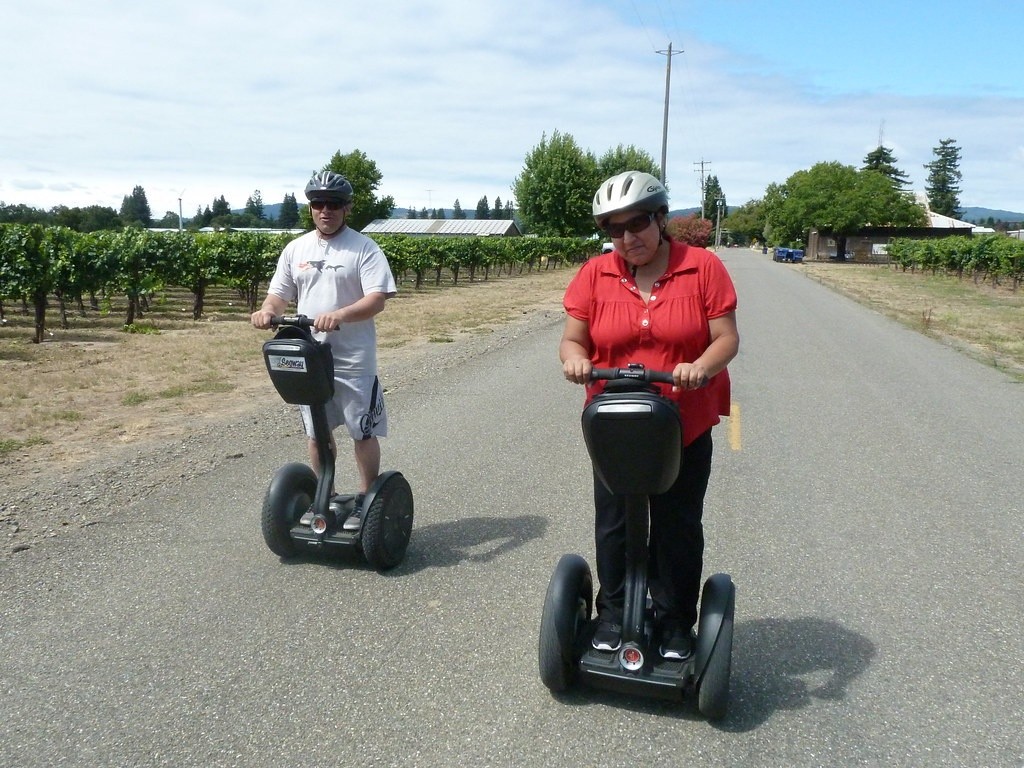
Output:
top-left (538, 363), bottom-right (735, 722)
top-left (253, 315), bottom-right (415, 570)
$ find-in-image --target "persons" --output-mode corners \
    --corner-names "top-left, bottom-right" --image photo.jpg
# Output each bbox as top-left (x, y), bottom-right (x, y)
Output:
top-left (249, 169), bottom-right (400, 533)
top-left (559, 170), bottom-right (739, 662)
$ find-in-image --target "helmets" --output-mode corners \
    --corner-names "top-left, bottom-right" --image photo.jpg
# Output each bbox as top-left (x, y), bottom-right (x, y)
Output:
top-left (305, 171), bottom-right (353, 201)
top-left (592, 171), bottom-right (669, 229)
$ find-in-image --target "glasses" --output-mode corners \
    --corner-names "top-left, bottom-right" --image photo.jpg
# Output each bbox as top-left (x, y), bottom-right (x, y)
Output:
top-left (310, 201), bottom-right (343, 210)
top-left (604, 212), bottom-right (655, 237)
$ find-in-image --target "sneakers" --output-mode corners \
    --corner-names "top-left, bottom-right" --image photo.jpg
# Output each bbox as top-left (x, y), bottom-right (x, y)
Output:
top-left (657, 628), bottom-right (691, 660)
top-left (300, 500), bottom-right (315, 525)
top-left (342, 492), bottom-right (366, 530)
top-left (591, 619), bottom-right (621, 652)
